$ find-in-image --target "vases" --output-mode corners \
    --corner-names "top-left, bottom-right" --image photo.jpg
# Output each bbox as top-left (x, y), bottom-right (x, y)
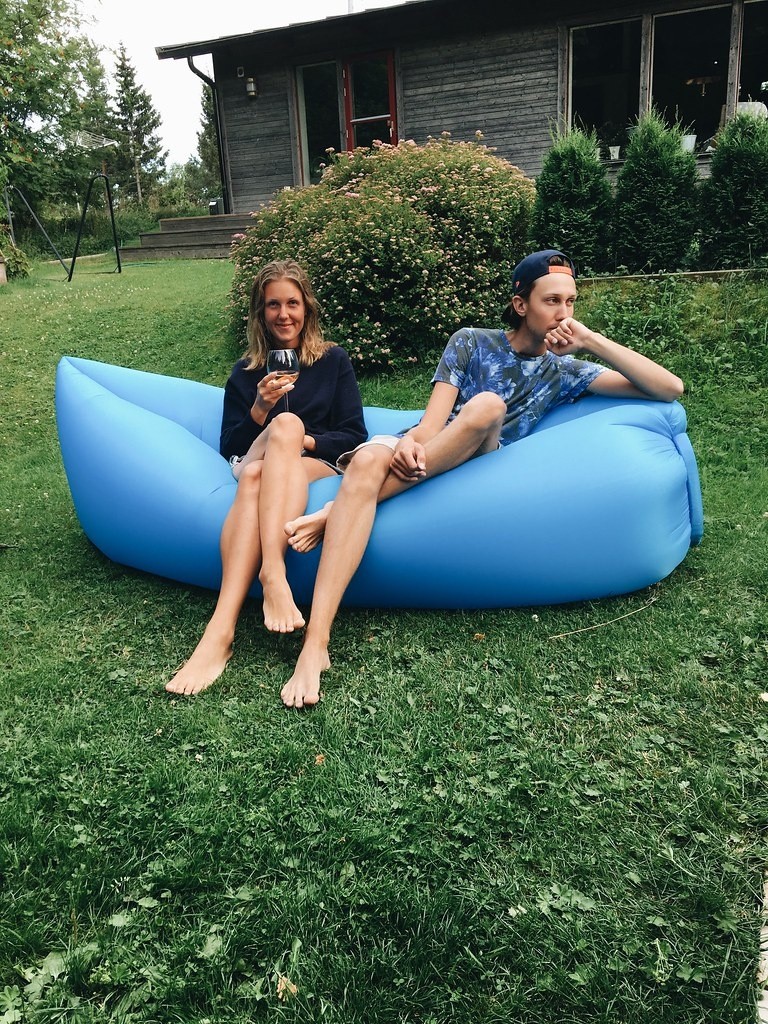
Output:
top-left (609, 146), bottom-right (621, 159)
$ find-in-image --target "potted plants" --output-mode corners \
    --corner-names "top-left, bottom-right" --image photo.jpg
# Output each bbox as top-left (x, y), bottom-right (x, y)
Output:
top-left (679, 127), bottom-right (697, 153)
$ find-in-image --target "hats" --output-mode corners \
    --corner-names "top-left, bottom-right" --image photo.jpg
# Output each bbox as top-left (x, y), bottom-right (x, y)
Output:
top-left (501, 250), bottom-right (576, 327)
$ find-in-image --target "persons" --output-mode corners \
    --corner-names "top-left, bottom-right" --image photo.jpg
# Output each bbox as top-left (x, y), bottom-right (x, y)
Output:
top-left (281, 250), bottom-right (684, 708)
top-left (164, 257), bottom-right (368, 696)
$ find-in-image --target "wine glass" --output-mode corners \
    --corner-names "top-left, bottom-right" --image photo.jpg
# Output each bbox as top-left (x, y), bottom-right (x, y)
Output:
top-left (268, 350), bottom-right (300, 413)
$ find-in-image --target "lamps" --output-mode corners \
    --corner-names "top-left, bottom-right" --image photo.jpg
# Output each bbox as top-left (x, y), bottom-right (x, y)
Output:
top-left (246, 77), bottom-right (258, 96)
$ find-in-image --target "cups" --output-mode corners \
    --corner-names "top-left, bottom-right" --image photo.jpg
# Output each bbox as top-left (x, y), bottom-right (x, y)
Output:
top-left (609, 146), bottom-right (620, 160)
top-left (681, 135), bottom-right (696, 154)
